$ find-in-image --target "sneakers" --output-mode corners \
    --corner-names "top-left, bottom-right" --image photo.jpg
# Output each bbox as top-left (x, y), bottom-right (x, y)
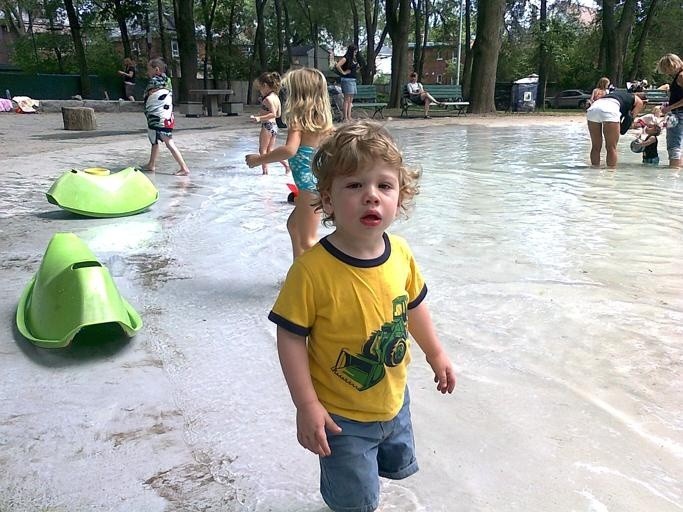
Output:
top-left (438, 103), bottom-right (445, 108)
top-left (423, 114), bottom-right (433, 120)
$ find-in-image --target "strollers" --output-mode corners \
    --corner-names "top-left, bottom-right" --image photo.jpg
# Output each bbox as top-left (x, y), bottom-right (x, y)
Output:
top-left (325, 79), bottom-right (346, 123)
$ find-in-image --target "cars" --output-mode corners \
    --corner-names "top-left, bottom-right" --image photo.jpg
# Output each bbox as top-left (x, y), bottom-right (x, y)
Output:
top-left (544, 88), bottom-right (591, 110)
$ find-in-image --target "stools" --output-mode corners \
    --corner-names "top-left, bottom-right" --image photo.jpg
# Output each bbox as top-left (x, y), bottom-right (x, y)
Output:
top-left (177, 100), bottom-right (204, 119)
top-left (220, 100), bottom-right (243, 117)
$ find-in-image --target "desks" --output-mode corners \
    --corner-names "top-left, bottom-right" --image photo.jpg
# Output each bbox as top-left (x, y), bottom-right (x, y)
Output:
top-left (188, 89), bottom-right (234, 117)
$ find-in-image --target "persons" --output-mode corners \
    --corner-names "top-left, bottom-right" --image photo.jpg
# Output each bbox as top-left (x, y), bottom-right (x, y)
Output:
top-left (252, 70), bottom-right (289, 176)
top-left (117, 58), bottom-right (137, 102)
top-left (140, 58), bottom-right (189, 176)
top-left (406, 72), bottom-right (446, 120)
top-left (245, 68), bottom-right (335, 261)
top-left (268, 117), bottom-right (456, 511)
top-left (586, 53), bottom-right (683, 168)
top-left (335, 44), bottom-right (362, 123)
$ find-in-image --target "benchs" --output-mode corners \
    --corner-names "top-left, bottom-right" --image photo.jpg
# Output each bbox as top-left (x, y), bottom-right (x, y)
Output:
top-left (642, 89), bottom-right (670, 107)
top-left (338, 84), bottom-right (387, 120)
top-left (398, 83), bottom-right (470, 117)
